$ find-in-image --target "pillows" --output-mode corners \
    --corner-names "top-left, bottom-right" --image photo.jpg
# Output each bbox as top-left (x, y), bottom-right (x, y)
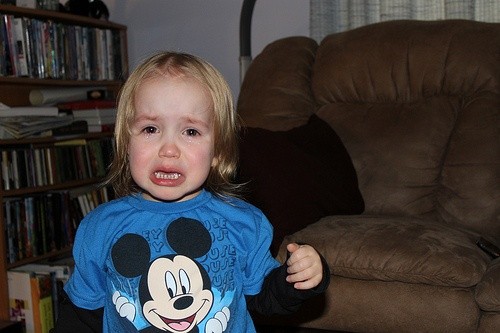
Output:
top-left (236, 110), bottom-right (365, 257)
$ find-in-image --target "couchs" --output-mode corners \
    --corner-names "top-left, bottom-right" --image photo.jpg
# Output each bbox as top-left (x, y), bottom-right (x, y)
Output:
top-left (235, 18), bottom-right (500, 333)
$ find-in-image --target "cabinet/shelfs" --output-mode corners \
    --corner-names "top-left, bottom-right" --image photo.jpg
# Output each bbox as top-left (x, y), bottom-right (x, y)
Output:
top-left (0, 3), bottom-right (127, 332)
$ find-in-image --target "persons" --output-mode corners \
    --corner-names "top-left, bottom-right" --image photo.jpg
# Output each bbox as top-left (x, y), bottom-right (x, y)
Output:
top-left (48, 51), bottom-right (331, 333)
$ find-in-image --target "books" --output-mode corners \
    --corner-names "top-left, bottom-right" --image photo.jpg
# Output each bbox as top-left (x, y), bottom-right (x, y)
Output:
top-left (0, 10), bottom-right (129, 333)
